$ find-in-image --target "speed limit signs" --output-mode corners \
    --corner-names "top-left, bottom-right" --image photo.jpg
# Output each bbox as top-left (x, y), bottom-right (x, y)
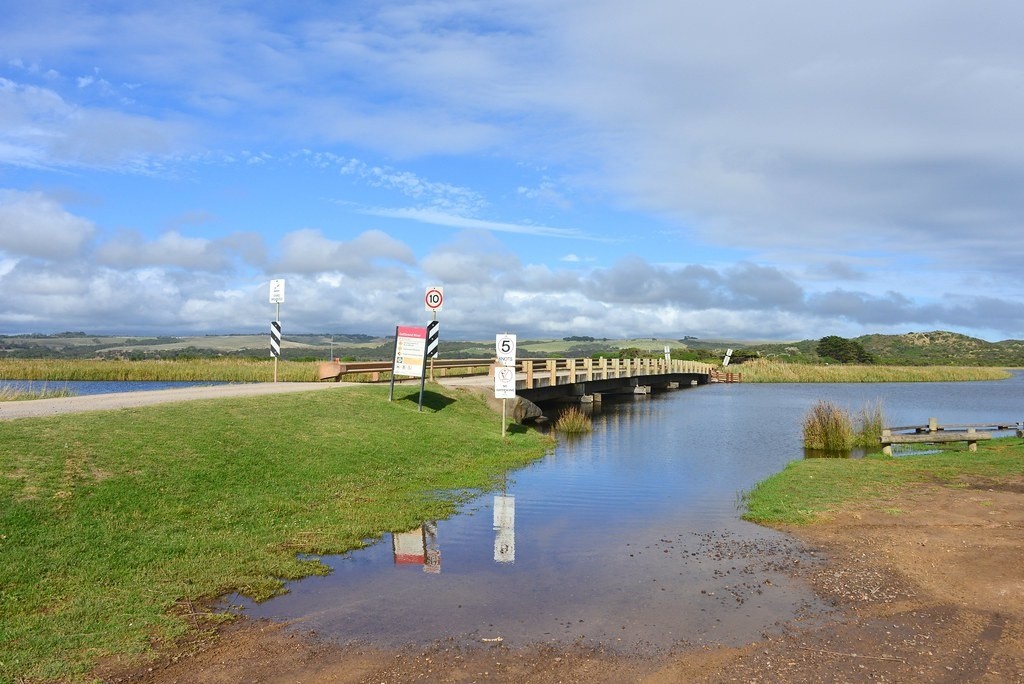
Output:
top-left (425, 286), bottom-right (443, 312)
top-left (423, 549), bottom-right (441, 574)
